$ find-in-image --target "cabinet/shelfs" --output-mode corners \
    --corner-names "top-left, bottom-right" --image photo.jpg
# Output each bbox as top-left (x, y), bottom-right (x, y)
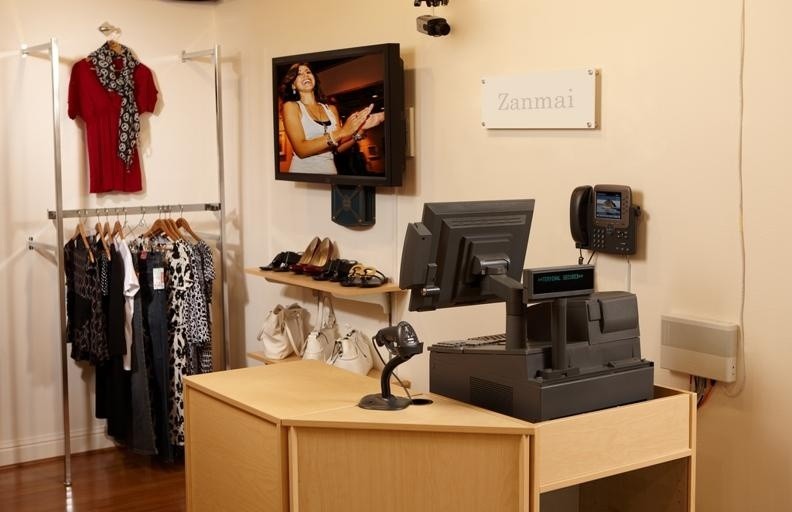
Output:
top-left (241, 261), bottom-right (412, 393)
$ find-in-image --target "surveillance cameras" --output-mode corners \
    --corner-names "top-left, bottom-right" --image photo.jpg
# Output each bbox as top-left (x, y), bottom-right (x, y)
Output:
top-left (416, 14), bottom-right (450, 37)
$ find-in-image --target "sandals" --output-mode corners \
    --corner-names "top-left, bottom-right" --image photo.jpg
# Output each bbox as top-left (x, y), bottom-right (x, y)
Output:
top-left (359, 265), bottom-right (388, 288)
top-left (342, 262), bottom-right (366, 287)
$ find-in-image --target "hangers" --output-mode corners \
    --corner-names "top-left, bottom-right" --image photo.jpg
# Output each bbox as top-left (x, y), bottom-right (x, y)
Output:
top-left (100, 25), bottom-right (131, 56)
top-left (65, 205), bottom-right (202, 262)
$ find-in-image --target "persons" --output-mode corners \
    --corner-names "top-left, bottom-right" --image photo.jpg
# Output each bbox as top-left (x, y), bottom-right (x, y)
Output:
top-left (277, 63), bottom-right (387, 177)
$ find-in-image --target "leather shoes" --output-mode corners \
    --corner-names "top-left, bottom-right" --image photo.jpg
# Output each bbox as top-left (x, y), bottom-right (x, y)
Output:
top-left (330, 258), bottom-right (358, 283)
top-left (289, 235), bottom-right (321, 273)
top-left (313, 257), bottom-right (343, 281)
top-left (272, 251), bottom-right (301, 272)
top-left (303, 236), bottom-right (334, 274)
top-left (259, 250), bottom-right (286, 271)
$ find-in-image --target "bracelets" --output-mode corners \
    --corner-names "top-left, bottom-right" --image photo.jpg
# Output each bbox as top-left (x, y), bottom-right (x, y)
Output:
top-left (326, 132), bottom-right (339, 148)
top-left (354, 132), bottom-right (362, 141)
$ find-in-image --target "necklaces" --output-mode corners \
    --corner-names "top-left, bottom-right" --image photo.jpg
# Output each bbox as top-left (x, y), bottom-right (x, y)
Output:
top-left (305, 104), bottom-right (328, 134)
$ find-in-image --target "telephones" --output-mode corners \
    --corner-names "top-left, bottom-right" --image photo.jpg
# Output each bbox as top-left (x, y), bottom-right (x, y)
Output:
top-left (570, 185), bottom-right (641, 255)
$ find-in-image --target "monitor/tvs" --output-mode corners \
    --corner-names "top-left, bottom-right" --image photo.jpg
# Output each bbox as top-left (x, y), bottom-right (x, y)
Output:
top-left (271, 43), bottom-right (405, 187)
top-left (399, 198), bottom-right (536, 311)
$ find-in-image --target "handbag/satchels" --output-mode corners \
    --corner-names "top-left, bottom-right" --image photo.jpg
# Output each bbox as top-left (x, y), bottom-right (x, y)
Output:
top-left (257, 303), bottom-right (304, 360)
top-left (302, 326), bottom-right (335, 362)
top-left (334, 329), bottom-right (374, 377)
